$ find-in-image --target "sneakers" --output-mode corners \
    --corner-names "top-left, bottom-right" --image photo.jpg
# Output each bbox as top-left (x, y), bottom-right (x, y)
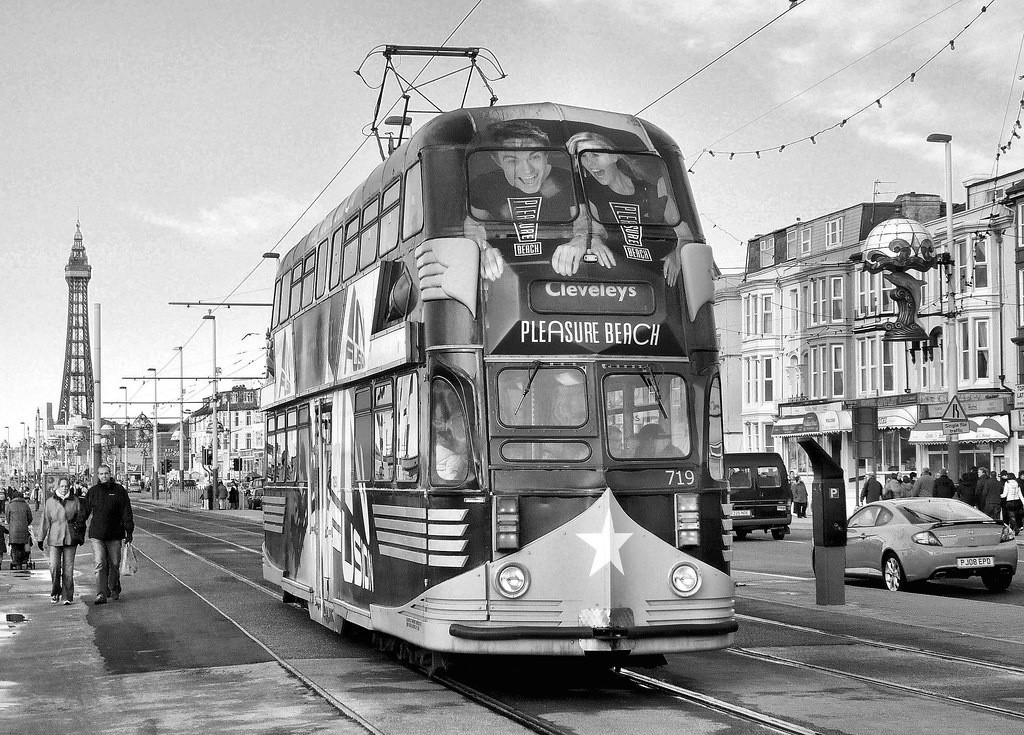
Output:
top-left (63, 598), bottom-right (72, 605)
top-left (51, 592), bottom-right (60, 603)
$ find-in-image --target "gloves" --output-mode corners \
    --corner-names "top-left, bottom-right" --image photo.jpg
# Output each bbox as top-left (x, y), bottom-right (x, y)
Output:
top-left (75, 530), bottom-right (85, 545)
top-left (38, 541), bottom-right (44, 551)
top-left (123, 533), bottom-right (132, 544)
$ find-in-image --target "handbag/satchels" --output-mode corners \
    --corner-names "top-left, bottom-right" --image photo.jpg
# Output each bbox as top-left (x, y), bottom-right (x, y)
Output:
top-left (121, 542), bottom-right (138, 575)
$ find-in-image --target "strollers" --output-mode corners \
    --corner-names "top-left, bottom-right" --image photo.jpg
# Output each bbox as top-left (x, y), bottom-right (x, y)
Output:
top-left (10, 532), bottom-right (35, 571)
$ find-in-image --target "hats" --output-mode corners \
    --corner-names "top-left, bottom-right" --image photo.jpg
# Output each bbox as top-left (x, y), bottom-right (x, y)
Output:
top-left (639, 425), bottom-right (670, 441)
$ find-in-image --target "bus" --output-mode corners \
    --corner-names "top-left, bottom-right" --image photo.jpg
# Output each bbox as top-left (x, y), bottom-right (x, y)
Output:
top-left (258, 43), bottom-right (740, 669)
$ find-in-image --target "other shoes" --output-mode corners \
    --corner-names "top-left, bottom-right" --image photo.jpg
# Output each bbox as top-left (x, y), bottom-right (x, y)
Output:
top-left (803, 515), bottom-right (807, 518)
top-left (798, 514), bottom-right (802, 518)
top-left (1015, 529), bottom-right (1019, 535)
top-left (106, 587), bottom-right (111, 597)
top-left (95, 593), bottom-right (107, 604)
top-left (112, 589), bottom-right (119, 600)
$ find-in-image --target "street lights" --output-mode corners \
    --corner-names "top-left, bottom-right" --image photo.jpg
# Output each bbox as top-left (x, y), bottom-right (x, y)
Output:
top-left (927, 132), bottom-right (961, 502)
top-left (203, 314), bottom-right (219, 511)
top-left (172, 345), bottom-right (184, 493)
top-left (20, 421), bottom-right (25, 477)
top-left (4, 426), bottom-right (9, 473)
top-left (147, 368), bottom-right (160, 500)
top-left (119, 386), bottom-right (129, 493)
top-left (262, 252), bottom-right (280, 271)
top-left (383, 115), bottom-right (413, 140)
top-left (59, 409), bottom-right (68, 468)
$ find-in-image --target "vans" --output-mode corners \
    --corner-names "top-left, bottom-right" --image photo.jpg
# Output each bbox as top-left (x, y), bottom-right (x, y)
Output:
top-left (725, 452), bottom-right (793, 541)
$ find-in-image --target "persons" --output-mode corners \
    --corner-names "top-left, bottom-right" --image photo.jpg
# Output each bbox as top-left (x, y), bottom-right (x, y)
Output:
top-left (860, 465), bottom-right (1024, 535)
top-left (70, 481), bottom-right (88, 497)
top-left (608, 423), bottom-right (685, 458)
top-left (200, 481), bottom-right (239, 510)
top-left (37, 477), bottom-right (81, 605)
top-left (464, 123), bottom-right (587, 281)
top-left (74, 465), bottom-right (135, 604)
top-left (792, 476), bottom-right (808, 518)
top-left (566, 131), bottom-right (694, 287)
top-left (0, 483), bottom-right (42, 568)
top-left (787, 471), bottom-right (797, 513)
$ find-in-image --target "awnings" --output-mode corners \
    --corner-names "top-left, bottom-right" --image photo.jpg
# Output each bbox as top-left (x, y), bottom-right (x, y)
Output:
top-left (771, 407), bottom-right (927, 437)
top-left (908, 415), bottom-right (1010, 445)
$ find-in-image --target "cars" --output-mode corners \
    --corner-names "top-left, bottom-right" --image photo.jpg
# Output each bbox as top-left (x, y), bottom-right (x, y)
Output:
top-left (115, 481), bottom-right (141, 493)
top-left (184, 480), bottom-right (198, 489)
top-left (810, 496), bottom-right (1019, 595)
top-left (224, 480), bottom-right (250, 496)
top-left (159, 479), bottom-right (178, 493)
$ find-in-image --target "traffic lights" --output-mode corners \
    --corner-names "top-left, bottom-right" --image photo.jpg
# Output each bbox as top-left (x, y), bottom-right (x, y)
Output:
top-left (165, 459), bottom-right (173, 473)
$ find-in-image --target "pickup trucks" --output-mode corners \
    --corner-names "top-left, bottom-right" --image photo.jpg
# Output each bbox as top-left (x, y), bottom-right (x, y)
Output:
top-left (245, 478), bottom-right (263, 511)
